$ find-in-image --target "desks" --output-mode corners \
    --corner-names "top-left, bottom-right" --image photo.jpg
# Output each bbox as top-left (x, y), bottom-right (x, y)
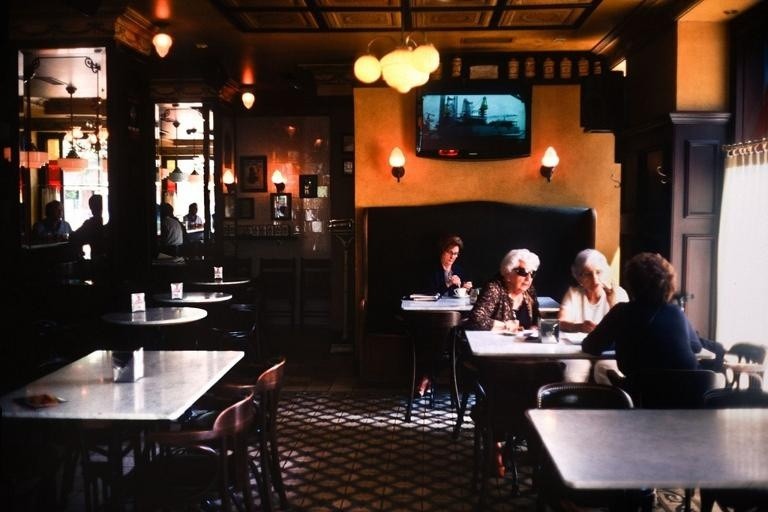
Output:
top-left (523, 403), bottom-right (768, 511)
top-left (221, 237), bottom-right (305, 327)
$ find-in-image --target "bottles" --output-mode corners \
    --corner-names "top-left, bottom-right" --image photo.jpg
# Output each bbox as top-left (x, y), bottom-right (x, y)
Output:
top-left (469, 287), bottom-right (480, 305)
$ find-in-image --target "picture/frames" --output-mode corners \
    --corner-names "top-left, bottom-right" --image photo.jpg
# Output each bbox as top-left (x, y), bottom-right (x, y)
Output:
top-left (296, 174), bottom-right (319, 199)
top-left (240, 155), bottom-right (268, 194)
top-left (269, 193), bottom-right (291, 221)
top-left (235, 197), bottom-right (254, 219)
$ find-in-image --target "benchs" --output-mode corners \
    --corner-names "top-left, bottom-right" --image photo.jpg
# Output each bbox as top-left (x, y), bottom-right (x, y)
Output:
top-left (362, 202), bottom-right (595, 398)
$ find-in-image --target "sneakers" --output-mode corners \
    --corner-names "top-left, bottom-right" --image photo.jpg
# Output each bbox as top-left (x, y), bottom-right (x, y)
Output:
top-left (427, 384), bottom-right (434, 396)
top-left (415, 381), bottom-right (426, 397)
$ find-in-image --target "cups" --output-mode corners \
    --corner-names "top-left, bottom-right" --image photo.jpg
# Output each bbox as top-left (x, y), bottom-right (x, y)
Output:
top-left (453, 288), bottom-right (466, 296)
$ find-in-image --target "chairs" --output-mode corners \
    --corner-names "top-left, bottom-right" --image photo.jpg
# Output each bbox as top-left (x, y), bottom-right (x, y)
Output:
top-left (398, 288), bottom-right (767, 488)
top-left (697, 383), bottom-right (767, 512)
top-left (1, 272), bottom-right (292, 512)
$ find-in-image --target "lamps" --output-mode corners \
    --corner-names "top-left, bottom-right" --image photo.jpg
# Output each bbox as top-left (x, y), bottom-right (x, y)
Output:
top-left (346, 2), bottom-right (445, 96)
top-left (240, 85), bottom-right (260, 113)
top-left (187, 129), bottom-right (202, 184)
top-left (150, 18), bottom-right (175, 63)
top-left (82, 55), bottom-right (108, 161)
top-left (389, 144), bottom-right (408, 182)
top-left (538, 143), bottom-right (560, 184)
top-left (55, 82), bottom-right (89, 174)
top-left (271, 169), bottom-right (285, 192)
top-left (167, 122), bottom-right (186, 185)
top-left (223, 171), bottom-right (234, 195)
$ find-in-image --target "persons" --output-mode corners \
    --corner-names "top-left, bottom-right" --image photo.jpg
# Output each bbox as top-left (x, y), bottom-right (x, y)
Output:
top-left (32, 201), bottom-right (71, 244)
top-left (582, 252), bottom-right (703, 378)
top-left (461, 247), bottom-right (541, 478)
top-left (75, 195), bottom-right (103, 232)
top-left (408, 234), bottom-right (481, 398)
top-left (140, 204), bottom-right (184, 257)
top-left (557, 249), bottom-right (631, 400)
top-left (183, 203), bottom-right (202, 230)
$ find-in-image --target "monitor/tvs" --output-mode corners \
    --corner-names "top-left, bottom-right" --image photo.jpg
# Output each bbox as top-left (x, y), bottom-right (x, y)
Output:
top-left (416, 77), bottom-right (532, 162)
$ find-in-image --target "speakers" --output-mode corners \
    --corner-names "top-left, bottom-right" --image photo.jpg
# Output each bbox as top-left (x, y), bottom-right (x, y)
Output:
top-left (580, 71), bottom-right (624, 132)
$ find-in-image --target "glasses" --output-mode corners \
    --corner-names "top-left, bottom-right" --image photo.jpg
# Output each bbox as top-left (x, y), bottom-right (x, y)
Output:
top-left (515, 266), bottom-right (536, 278)
